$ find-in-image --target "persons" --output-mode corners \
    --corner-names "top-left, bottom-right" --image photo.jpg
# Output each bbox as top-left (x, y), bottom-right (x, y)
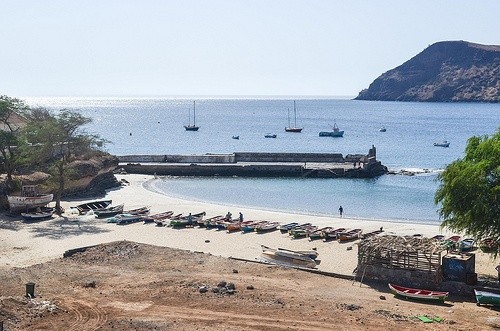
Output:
top-left (226, 212), bottom-right (232, 221)
top-left (339, 206), bottom-right (344, 215)
top-left (188, 213), bottom-right (193, 226)
top-left (239, 212), bottom-right (243, 222)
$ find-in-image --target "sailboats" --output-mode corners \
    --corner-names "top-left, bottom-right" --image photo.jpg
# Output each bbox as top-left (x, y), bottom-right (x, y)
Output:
top-left (284, 100), bottom-right (304, 132)
top-left (183, 100), bottom-right (200, 131)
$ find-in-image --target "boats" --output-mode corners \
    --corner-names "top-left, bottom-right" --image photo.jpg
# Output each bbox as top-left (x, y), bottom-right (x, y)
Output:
top-left (473, 288), bottom-right (500, 311)
top-left (232, 135), bottom-right (239, 139)
top-left (433, 137), bottom-right (451, 148)
top-left (106, 205), bottom-right (500, 270)
top-left (379, 127), bottom-right (387, 132)
top-left (76, 199), bottom-right (114, 213)
top-left (264, 134), bottom-right (277, 138)
top-left (90, 202), bottom-right (125, 219)
top-left (319, 122), bottom-right (345, 137)
top-left (6, 183), bottom-right (54, 213)
top-left (388, 282), bottom-right (450, 305)
top-left (20, 210), bottom-right (55, 221)
